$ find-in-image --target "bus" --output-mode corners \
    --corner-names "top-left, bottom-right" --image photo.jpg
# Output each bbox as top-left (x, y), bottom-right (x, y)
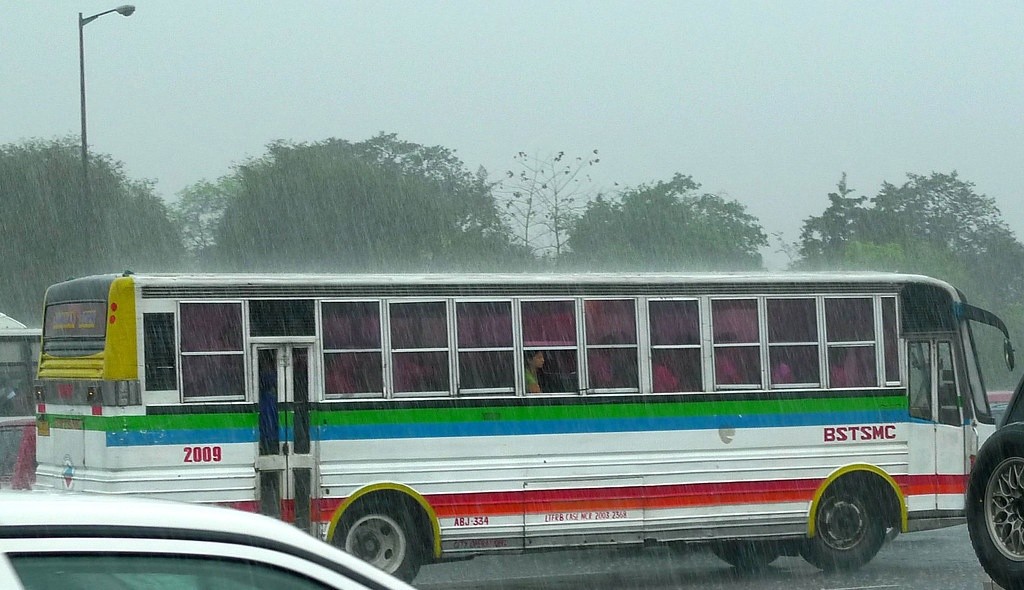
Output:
top-left (30, 266), bottom-right (1016, 589)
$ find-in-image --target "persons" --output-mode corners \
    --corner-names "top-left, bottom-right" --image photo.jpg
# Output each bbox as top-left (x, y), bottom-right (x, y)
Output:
top-left (260, 348), bottom-right (310, 526)
top-left (524, 352), bottom-right (545, 393)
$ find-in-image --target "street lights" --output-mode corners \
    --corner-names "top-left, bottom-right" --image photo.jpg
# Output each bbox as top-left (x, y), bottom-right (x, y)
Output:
top-left (76, 4), bottom-right (137, 278)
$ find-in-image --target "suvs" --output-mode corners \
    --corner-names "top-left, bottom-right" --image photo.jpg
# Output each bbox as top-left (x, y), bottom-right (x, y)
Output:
top-left (962, 365), bottom-right (1024, 590)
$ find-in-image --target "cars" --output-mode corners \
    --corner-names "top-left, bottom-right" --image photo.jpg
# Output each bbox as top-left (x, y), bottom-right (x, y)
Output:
top-left (0, 487), bottom-right (418, 589)
top-left (0, 414), bottom-right (38, 489)
